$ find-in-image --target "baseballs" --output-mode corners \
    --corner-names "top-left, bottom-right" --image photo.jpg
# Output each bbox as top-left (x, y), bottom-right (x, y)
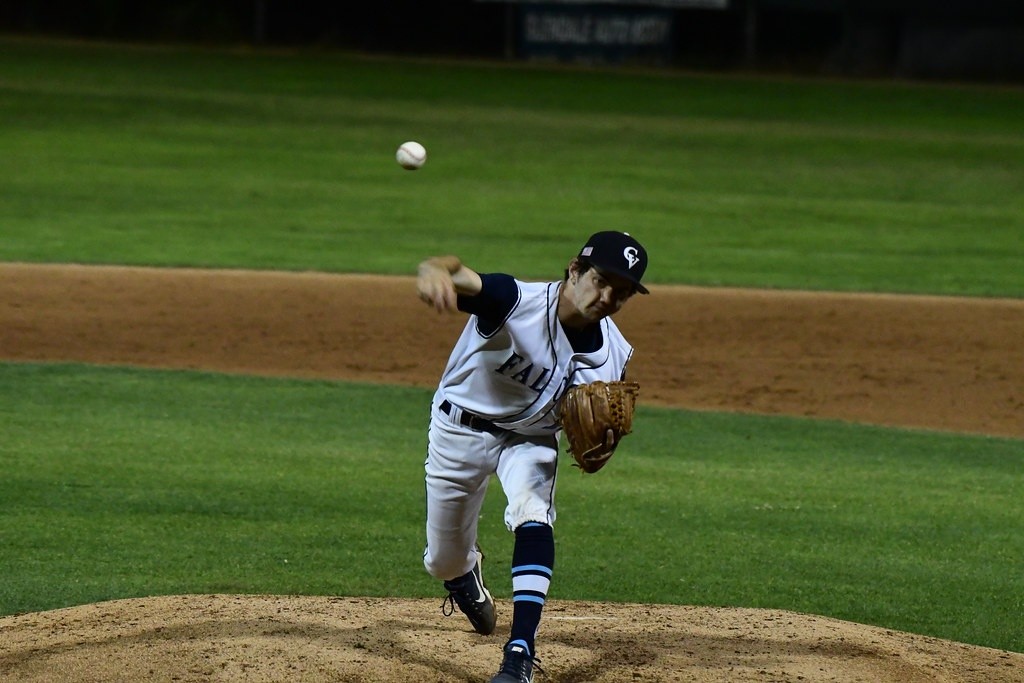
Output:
top-left (395, 140), bottom-right (427, 171)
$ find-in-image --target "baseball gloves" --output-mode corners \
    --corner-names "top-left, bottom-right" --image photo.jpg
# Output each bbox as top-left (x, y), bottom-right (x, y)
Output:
top-left (558, 380), bottom-right (641, 474)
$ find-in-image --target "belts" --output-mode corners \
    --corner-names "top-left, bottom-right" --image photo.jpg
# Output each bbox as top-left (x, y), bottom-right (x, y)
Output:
top-left (439, 399), bottom-right (503, 433)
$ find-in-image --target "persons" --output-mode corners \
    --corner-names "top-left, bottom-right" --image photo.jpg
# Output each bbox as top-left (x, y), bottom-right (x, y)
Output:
top-left (414, 229), bottom-right (649, 683)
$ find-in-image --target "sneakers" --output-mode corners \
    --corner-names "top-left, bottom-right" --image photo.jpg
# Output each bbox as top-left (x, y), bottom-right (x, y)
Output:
top-left (442, 544), bottom-right (497, 635)
top-left (490, 638), bottom-right (544, 683)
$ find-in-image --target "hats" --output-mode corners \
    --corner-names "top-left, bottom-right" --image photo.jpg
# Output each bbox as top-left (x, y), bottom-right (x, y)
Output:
top-left (577, 231), bottom-right (650, 294)
